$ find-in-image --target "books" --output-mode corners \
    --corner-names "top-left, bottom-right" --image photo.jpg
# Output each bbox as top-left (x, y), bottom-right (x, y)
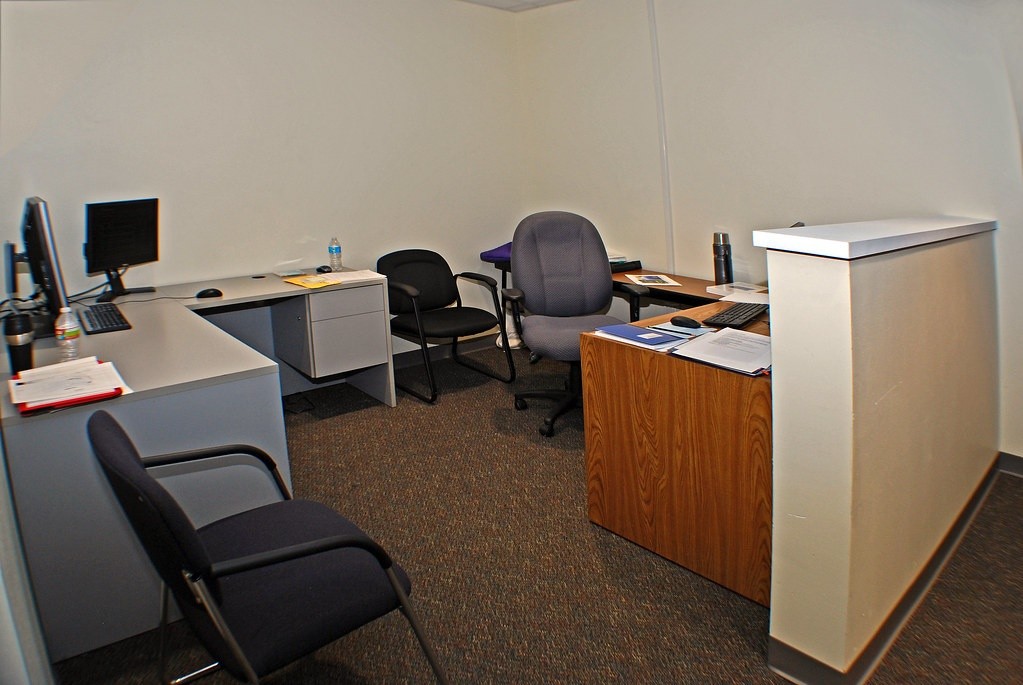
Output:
top-left (706, 281), bottom-right (768, 296)
top-left (595, 324), bottom-right (688, 352)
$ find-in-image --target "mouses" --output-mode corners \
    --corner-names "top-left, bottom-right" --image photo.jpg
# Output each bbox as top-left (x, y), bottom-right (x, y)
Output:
top-left (196, 288), bottom-right (222, 298)
top-left (316, 265), bottom-right (332, 273)
top-left (670, 316), bottom-right (702, 329)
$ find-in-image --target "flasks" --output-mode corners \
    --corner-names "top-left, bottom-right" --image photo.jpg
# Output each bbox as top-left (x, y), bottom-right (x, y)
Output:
top-left (3, 314), bottom-right (34, 375)
top-left (713, 232), bottom-right (733, 285)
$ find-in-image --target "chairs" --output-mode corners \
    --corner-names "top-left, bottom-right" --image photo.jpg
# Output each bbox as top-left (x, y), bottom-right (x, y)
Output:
top-left (377, 211), bottom-right (650, 436)
top-left (86, 410), bottom-right (449, 685)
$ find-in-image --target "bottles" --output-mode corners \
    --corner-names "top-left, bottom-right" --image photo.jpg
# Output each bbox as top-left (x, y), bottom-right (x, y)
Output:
top-left (328, 237), bottom-right (342, 271)
top-left (56, 307), bottom-right (81, 362)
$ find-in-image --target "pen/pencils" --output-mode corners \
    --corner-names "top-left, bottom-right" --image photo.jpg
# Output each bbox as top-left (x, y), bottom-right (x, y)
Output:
top-left (648, 326), bottom-right (696, 336)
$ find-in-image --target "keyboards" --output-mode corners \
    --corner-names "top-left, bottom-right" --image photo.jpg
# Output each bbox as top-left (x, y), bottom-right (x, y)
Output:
top-left (75, 302), bottom-right (132, 334)
top-left (702, 303), bottom-right (769, 329)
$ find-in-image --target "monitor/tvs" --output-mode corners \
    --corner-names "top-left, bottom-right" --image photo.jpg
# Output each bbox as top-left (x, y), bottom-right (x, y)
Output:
top-left (2, 197), bottom-right (69, 340)
top-left (82, 198), bottom-right (159, 302)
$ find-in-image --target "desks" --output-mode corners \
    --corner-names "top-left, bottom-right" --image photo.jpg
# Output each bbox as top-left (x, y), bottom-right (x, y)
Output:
top-left (0, 266), bottom-right (397, 664)
top-left (480, 240), bottom-right (772, 609)
